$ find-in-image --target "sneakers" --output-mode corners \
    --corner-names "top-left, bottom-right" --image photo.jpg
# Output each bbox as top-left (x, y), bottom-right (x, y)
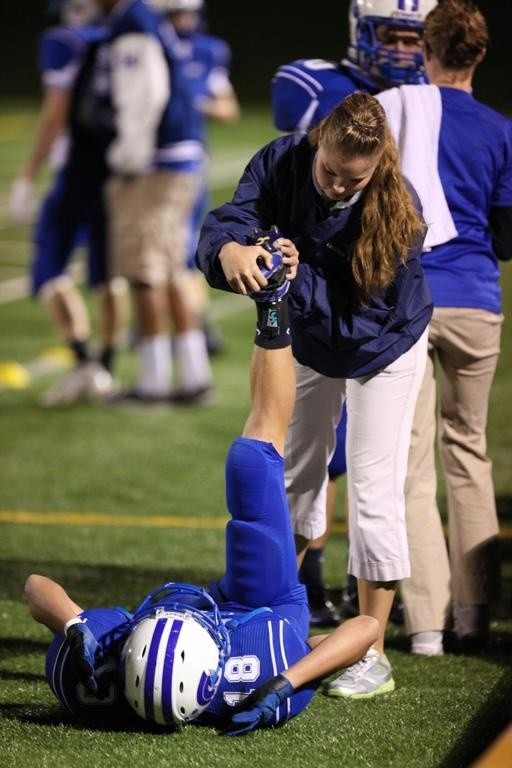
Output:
top-left (409, 630), bottom-right (441, 656)
top-left (327, 647), bottom-right (394, 699)
top-left (39, 345), bottom-right (210, 408)
top-left (241, 226), bottom-right (290, 303)
top-left (453, 601), bottom-right (484, 635)
top-left (309, 602), bottom-right (341, 627)
top-left (340, 595), bottom-right (405, 626)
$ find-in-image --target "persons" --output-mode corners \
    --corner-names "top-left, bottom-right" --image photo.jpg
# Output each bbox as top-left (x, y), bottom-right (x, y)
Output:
top-left (22, 217), bottom-right (382, 740)
top-left (86, 0), bottom-right (213, 404)
top-left (8, 0), bottom-right (129, 409)
top-left (121, 0), bottom-right (243, 352)
top-left (191, 88), bottom-right (436, 703)
top-left (372, 2), bottom-right (512, 660)
top-left (261, 2), bottom-right (450, 632)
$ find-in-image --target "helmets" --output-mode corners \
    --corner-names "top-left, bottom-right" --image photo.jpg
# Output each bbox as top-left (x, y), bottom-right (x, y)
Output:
top-left (340, 1), bottom-right (438, 84)
top-left (120, 582), bottom-right (230, 728)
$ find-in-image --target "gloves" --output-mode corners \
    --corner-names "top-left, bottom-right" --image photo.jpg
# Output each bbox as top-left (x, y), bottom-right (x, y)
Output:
top-left (226, 674), bottom-right (293, 736)
top-left (10, 180), bottom-right (33, 217)
top-left (63, 621), bottom-right (104, 687)
